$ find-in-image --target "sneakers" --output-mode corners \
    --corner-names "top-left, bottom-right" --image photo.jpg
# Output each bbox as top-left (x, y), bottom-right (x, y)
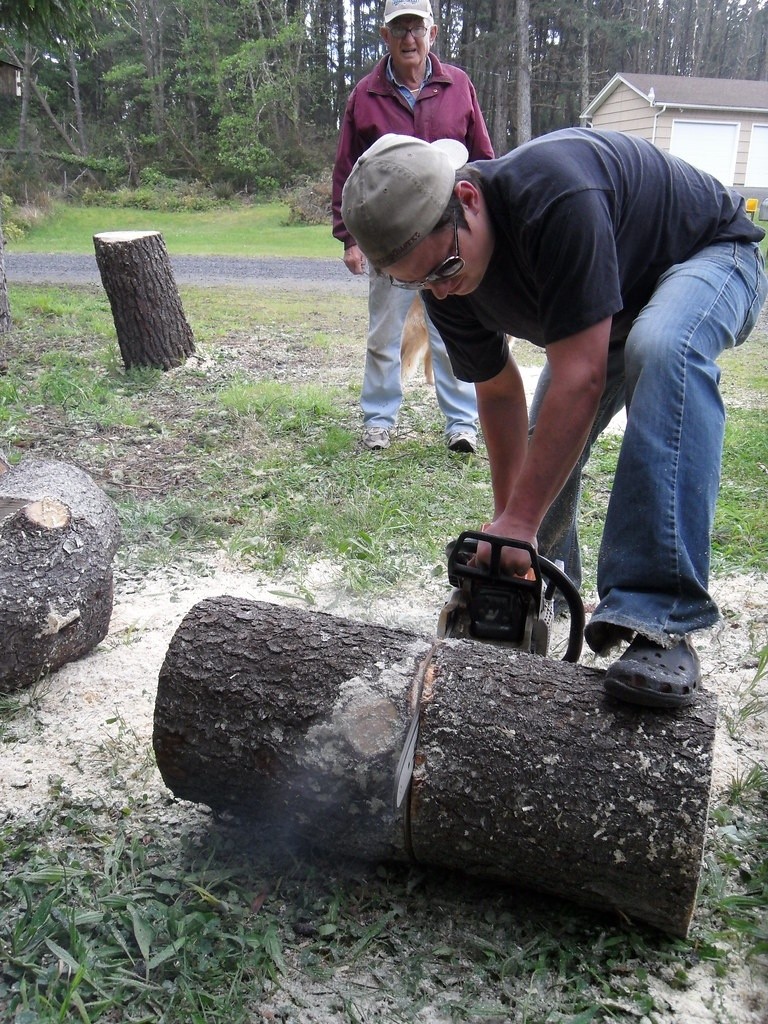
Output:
top-left (361, 426), bottom-right (391, 450)
top-left (446, 432), bottom-right (479, 456)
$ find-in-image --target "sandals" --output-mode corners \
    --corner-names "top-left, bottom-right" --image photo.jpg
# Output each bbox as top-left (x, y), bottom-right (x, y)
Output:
top-left (602, 631), bottom-right (703, 706)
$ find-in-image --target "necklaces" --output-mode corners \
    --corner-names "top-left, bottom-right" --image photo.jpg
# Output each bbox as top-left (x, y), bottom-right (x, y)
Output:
top-left (410, 88), bottom-right (420, 92)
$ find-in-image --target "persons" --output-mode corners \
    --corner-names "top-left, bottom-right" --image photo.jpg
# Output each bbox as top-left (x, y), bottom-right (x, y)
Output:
top-left (331, 0), bottom-right (494, 452)
top-left (343, 126), bottom-right (768, 706)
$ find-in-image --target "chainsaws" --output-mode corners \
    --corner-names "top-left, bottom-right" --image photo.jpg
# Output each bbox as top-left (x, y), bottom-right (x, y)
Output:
top-left (390, 523), bottom-right (588, 815)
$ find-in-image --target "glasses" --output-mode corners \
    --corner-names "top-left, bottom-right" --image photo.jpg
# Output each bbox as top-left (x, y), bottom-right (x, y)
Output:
top-left (386, 25), bottom-right (430, 39)
top-left (388, 209), bottom-right (465, 290)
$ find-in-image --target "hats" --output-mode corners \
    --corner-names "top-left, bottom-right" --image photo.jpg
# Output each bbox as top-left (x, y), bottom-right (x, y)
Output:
top-left (382, 0), bottom-right (434, 25)
top-left (340, 132), bottom-right (469, 271)
